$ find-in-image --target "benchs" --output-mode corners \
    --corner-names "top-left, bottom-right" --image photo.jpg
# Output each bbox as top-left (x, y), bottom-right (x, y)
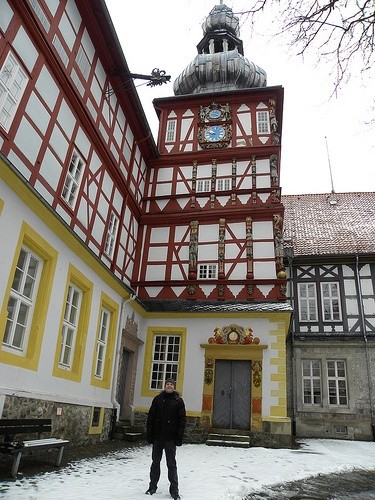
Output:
top-left (0, 418), bottom-right (70, 479)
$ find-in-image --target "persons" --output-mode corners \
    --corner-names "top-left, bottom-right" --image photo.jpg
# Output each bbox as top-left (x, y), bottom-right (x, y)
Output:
top-left (145, 378), bottom-right (186, 500)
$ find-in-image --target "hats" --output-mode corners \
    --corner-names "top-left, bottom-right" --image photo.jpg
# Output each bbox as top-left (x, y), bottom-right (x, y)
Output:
top-left (165, 378), bottom-right (175, 385)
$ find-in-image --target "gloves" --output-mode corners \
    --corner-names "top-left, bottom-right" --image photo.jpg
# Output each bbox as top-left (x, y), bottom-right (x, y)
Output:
top-left (147, 438), bottom-right (153, 444)
top-left (175, 438), bottom-right (182, 446)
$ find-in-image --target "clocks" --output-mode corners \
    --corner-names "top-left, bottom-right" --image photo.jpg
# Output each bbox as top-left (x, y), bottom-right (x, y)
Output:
top-left (205, 126), bottom-right (225, 142)
top-left (209, 110), bottom-right (222, 119)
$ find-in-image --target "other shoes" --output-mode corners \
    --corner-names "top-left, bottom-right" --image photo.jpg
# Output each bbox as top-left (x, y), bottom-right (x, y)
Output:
top-left (146, 489), bottom-right (156, 495)
top-left (171, 492), bottom-right (181, 500)
